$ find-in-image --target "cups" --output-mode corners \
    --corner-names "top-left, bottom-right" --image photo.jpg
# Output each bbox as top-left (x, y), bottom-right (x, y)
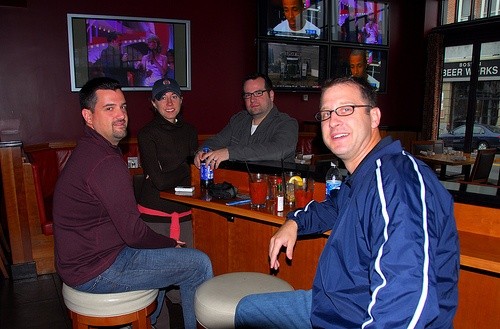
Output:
top-left (248, 174), bottom-right (269, 210)
top-left (294, 178), bottom-right (313, 209)
top-left (285, 171), bottom-right (301, 206)
top-left (427, 145), bottom-right (433, 158)
top-left (269, 172), bottom-right (284, 210)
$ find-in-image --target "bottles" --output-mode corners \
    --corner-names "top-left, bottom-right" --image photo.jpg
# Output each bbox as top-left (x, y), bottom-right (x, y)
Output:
top-left (276, 179), bottom-right (285, 212)
top-left (326, 163), bottom-right (342, 197)
top-left (200, 148), bottom-right (214, 202)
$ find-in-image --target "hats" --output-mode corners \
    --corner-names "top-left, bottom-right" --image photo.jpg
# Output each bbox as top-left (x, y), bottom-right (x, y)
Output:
top-left (152, 79), bottom-right (181, 100)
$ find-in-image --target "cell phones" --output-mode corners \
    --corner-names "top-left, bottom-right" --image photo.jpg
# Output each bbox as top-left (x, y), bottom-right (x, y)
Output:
top-left (175, 191), bottom-right (195, 196)
top-left (174, 186), bottom-right (195, 192)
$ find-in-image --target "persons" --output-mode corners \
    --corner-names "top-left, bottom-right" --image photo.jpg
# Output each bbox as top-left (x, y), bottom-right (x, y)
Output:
top-left (51, 77), bottom-right (215, 329)
top-left (193, 72), bottom-right (298, 170)
top-left (235, 75), bottom-right (460, 329)
top-left (267, 0), bottom-right (320, 39)
top-left (346, 49), bottom-right (380, 91)
top-left (342, 9), bottom-right (358, 42)
top-left (95, 32), bottom-right (175, 87)
top-left (361, 12), bottom-right (379, 44)
top-left (136, 77), bottom-right (198, 329)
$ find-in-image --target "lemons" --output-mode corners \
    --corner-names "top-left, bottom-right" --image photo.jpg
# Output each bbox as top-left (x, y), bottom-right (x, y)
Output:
top-left (288, 176), bottom-right (305, 187)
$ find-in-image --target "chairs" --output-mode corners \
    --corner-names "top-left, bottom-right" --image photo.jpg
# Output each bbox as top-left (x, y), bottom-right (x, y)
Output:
top-left (459, 149), bottom-right (496, 184)
top-left (409, 139), bottom-right (446, 169)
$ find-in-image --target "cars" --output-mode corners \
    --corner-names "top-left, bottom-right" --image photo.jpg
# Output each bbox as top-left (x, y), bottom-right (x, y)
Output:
top-left (439, 123), bottom-right (500, 151)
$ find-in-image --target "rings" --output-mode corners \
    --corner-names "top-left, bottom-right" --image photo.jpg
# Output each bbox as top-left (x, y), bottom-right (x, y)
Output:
top-left (213, 159), bottom-right (217, 161)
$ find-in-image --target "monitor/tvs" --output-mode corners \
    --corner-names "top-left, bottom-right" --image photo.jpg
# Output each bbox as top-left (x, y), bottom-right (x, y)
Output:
top-left (66, 13), bottom-right (191, 91)
top-left (256, 0), bottom-right (391, 95)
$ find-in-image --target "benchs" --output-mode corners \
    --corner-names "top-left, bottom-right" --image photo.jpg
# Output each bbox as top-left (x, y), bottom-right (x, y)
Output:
top-left (0, 133), bottom-right (214, 276)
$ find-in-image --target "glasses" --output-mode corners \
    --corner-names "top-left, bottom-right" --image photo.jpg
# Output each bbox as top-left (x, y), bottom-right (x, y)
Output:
top-left (242, 88), bottom-right (272, 99)
top-left (315, 105), bottom-right (374, 121)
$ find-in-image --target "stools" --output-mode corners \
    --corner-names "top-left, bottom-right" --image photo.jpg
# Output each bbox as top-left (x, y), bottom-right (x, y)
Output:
top-left (194, 271), bottom-right (295, 329)
top-left (62, 282), bottom-right (159, 329)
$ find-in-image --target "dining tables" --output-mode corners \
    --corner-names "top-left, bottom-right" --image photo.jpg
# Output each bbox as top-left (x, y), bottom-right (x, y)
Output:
top-left (421, 154), bottom-right (476, 180)
